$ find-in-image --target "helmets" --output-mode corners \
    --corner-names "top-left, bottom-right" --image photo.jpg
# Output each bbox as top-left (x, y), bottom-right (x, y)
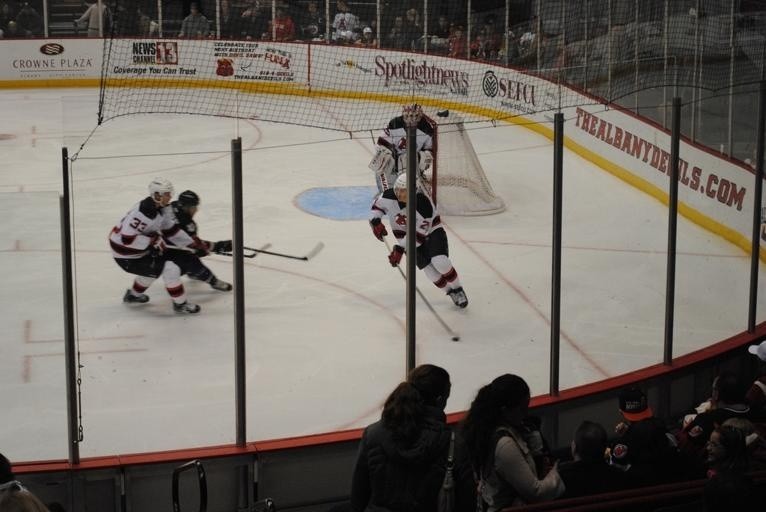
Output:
top-left (148, 178), bottom-right (174, 202)
top-left (402, 104), bottom-right (423, 122)
top-left (394, 172), bottom-right (419, 199)
top-left (179, 190), bottom-right (200, 207)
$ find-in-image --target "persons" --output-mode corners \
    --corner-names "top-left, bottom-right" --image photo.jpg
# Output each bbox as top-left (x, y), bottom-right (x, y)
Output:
top-left (1, 1), bottom-right (621, 65)
top-left (563, 341), bottom-right (766, 492)
top-left (108, 180), bottom-right (233, 315)
top-left (367, 174), bottom-right (470, 309)
top-left (0, 454), bottom-right (50, 512)
top-left (349, 364), bottom-right (453, 509)
top-left (452, 374), bottom-right (564, 509)
top-left (368, 104), bottom-right (435, 194)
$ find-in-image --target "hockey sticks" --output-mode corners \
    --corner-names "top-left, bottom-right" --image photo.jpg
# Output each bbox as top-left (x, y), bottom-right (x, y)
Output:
top-left (241, 242), bottom-right (324, 261)
top-left (382, 233), bottom-right (462, 342)
top-left (151, 242), bottom-right (269, 258)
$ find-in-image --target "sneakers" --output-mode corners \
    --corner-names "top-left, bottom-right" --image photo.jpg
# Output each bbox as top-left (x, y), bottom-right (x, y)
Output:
top-left (446, 287), bottom-right (469, 308)
top-left (213, 240), bottom-right (233, 251)
top-left (123, 289), bottom-right (149, 303)
top-left (173, 301), bottom-right (200, 313)
top-left (213, 281), bottom-right (233, 291)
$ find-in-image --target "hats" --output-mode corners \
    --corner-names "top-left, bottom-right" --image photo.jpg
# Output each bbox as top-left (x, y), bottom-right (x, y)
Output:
top-left (619, 388), bottom-right (653, 422)
top-left (748, 341), bottom-right (765, 360)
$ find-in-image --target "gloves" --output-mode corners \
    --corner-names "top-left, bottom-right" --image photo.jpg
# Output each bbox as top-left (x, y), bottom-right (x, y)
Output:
top-left (373, 223), bottom-right (387, 241)
top-left (389, 245), bottom-right (404, 266)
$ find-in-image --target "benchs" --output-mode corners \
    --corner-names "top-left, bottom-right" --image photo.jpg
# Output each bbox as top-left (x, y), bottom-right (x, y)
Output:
top-left (501, 469), bottom-right (766, 512)
top-left (0, 0), bottom-right (391, 50)
